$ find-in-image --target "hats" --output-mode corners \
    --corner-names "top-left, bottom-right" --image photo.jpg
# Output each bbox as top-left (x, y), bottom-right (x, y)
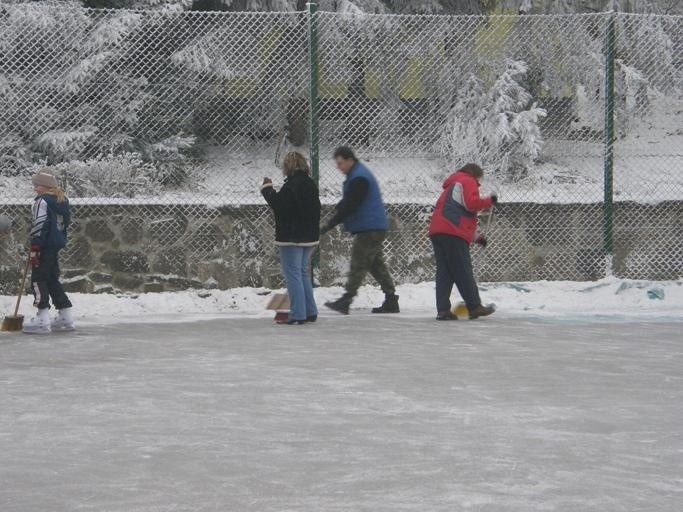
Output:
top-left (32, 168), bottom-right (58, 188)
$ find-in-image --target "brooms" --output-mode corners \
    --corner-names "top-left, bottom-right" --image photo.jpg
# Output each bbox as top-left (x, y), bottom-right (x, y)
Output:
top-left (0, 245), bottom-right (31, 331)
top-left (267, 233), bottom-right (324, 311)
top-left (273, 311), bottom-right (289, 324)
top-left (453, 204), bottom-right (495, 317)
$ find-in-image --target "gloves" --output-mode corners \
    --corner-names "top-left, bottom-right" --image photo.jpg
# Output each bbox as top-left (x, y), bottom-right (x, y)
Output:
top-left (30, 244), bottom-right (41, 268)
top-left (490, 192), bottom-right (498, 205)
top-left (476, 237), bottom-right (486, 247)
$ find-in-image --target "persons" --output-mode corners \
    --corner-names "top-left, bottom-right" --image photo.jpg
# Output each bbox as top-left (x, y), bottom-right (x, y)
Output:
top-left (428, 163), bottom-right (497, 320)
top-left (259, 151), bottom-right (320, 326)
top-left (317, 145), bottom-right (399, 315)
top-left (18, 171), bottom-right (75, 333)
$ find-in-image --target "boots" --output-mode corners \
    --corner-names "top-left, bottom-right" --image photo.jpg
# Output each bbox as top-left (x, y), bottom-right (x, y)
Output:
top-left (370, 293), bottom-right (401, 313)
top-left (49, 307), bottom-right (74, 327)
top-left (325, 293), bottom-right (353, 314)
top-left (22, 307), bottom-right (52, 329)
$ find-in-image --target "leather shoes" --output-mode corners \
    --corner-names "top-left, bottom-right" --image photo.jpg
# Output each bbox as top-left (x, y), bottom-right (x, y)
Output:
top-left (468, 302), bottom-right (497, 320)
top-left (435, 309), bottom-right (458, 321)
top-left (302, 314), bottom-right (317, 324)
top-left (275, 316), bottom-right (304, 326)
